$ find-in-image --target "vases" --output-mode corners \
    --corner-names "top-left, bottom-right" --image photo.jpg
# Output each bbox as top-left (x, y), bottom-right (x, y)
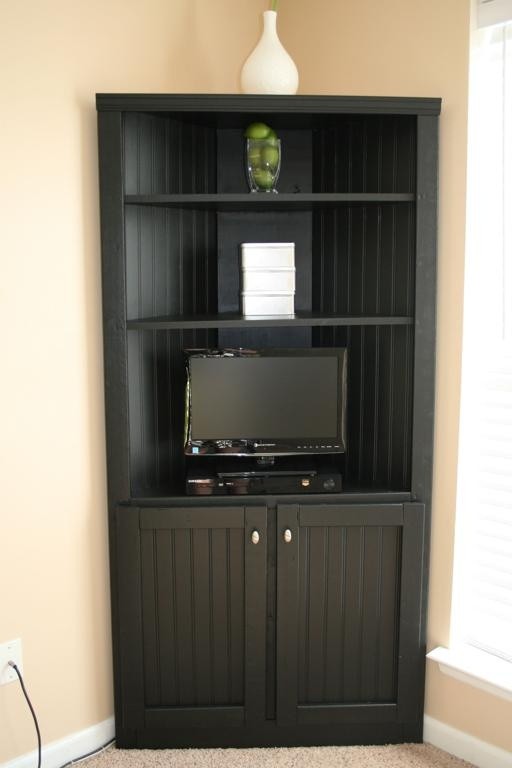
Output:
top-left (239, 9), bottom-right (300, 95)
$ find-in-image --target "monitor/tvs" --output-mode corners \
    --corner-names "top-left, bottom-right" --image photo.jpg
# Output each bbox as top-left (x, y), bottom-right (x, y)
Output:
top-left (183, 346), bottom-right (348, 478)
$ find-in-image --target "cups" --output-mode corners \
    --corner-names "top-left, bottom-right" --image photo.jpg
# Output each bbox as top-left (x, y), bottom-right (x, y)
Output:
top-left (247, 139), bottom-right (282, 195)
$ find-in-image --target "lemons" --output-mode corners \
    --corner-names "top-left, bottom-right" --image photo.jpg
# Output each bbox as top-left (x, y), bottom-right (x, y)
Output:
top-left (243, 123), bottom-right (280, 190)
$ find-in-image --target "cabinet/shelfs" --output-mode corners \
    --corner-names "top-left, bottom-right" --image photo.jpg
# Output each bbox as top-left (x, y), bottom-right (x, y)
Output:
top-left (96, 92), bottom-right (442, 751)
